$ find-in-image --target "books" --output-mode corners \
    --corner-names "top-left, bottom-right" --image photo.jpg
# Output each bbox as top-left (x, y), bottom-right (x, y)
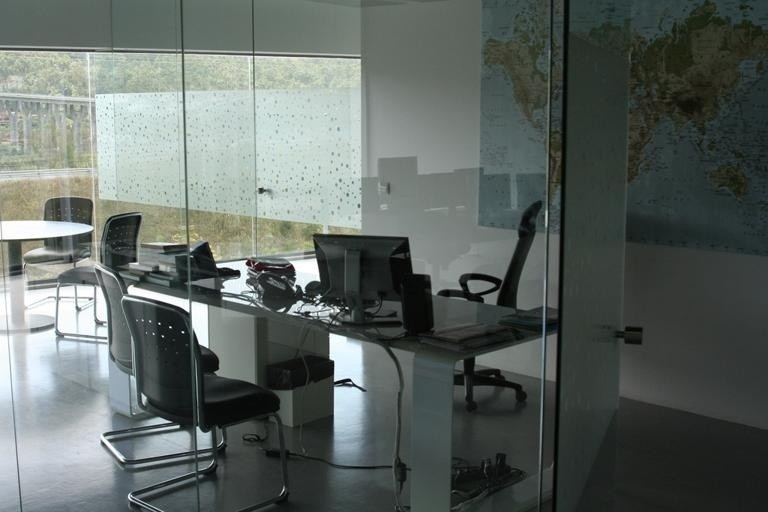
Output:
top-left (418, 304), bottom-right (559, 353)
top-left (117, 241), bottom-right (188, 288)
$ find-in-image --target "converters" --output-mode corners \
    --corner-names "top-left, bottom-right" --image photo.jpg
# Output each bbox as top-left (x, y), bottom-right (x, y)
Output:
top-left (266, 447), bottom-right (290, 458)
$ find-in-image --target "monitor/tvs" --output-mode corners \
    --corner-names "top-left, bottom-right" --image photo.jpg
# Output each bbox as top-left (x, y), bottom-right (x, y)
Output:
top-left (313, 233), bottom-right (414, 325)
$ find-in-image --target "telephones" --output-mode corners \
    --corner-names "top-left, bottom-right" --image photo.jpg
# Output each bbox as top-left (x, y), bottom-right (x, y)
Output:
top-left (245, 256), bottom-right (296, 276)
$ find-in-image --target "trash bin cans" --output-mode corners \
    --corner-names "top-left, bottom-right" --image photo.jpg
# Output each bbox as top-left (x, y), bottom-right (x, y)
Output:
top-left (270, 354), bottom-right (335, 428)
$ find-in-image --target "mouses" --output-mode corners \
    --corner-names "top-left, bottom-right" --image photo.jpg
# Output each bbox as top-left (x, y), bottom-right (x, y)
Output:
top-left (305, 280), bottom-right (321, 292)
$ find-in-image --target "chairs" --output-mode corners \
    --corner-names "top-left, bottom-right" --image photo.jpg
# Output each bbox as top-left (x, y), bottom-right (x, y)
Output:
top-left (22, 196), bottom-right (143, 340)
top-left (435, 200), bottom-right (544, 413)
top-left (120, 295), bottom-right (291, 511)
top-left (94, 262), bottom-right (227, 468)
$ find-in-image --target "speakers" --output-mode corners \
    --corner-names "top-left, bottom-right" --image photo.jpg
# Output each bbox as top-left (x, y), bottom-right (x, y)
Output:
top-left (401, 274), bottom-right (436, 334)
top-left (189, 240), bottom-right (218, 277)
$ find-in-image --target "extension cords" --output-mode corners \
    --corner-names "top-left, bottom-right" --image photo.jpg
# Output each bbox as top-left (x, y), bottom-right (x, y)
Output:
top-left (455, 462), bottom-right (521, 492)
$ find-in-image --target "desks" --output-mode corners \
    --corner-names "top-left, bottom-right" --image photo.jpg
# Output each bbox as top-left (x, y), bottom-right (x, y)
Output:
top-left (133, 272), bottom-right (559, 511)
top-left (1, 220), bottom-right (96, 335)
